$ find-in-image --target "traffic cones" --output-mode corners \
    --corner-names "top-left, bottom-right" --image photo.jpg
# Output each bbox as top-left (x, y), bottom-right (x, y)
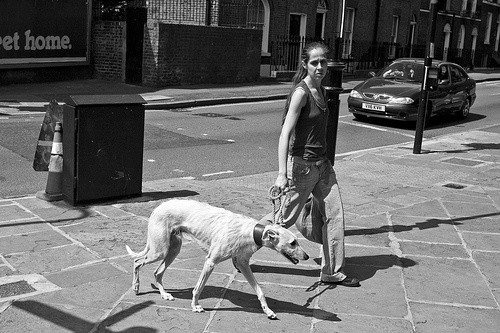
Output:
top-left (35, 122), bottom-right (65, 203)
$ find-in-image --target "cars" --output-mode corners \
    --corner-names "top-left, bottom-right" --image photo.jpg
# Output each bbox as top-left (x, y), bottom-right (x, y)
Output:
top-left (347, 57), bottom-right (477, 129)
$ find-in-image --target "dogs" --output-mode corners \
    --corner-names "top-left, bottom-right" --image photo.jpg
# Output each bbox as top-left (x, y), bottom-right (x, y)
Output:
top-left (125, 198), bottom-right (309, 320)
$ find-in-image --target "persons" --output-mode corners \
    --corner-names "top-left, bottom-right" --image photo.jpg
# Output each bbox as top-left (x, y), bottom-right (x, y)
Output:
top-left (273, 42), bottom-right (361, 285)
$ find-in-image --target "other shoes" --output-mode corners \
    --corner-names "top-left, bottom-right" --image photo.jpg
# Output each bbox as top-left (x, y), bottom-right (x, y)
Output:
top-left (321, 275), bottom-right (359, 286)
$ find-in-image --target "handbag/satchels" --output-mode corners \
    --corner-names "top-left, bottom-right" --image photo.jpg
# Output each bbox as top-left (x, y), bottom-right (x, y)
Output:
top-left (294, 195), bottom-right (323, 244)
